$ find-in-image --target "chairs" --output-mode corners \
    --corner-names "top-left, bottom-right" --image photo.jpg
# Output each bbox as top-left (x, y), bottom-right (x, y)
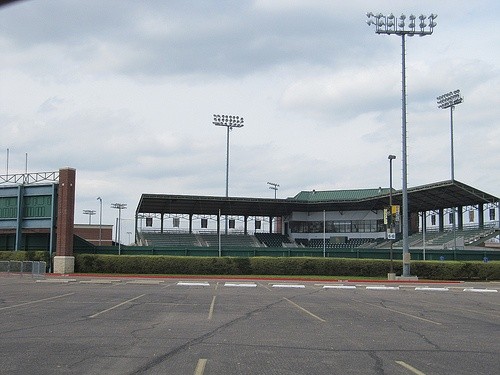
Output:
top-left (138, 229), bottom-right (500, 249)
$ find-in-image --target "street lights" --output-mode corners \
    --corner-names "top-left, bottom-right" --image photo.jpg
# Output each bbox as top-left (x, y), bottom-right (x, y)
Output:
top-left (387, 154), bottom-right (399, 282)
top-left (97, 196), bottom-right (102, 247)
top-left (126, 232), bottom-right (132, 246)
top-left (83, 209), bottom-right (96, 225)
top-left (366, 11), bottom-right (436, 277)
top-left (267, 182), bottom-right (280, 199)
top-left (436, 89), bottom-right (464, 261)
top-left (212, 113), bottom-right (245, 236)
top-left (110, 202), bottom-right (128, 256)
